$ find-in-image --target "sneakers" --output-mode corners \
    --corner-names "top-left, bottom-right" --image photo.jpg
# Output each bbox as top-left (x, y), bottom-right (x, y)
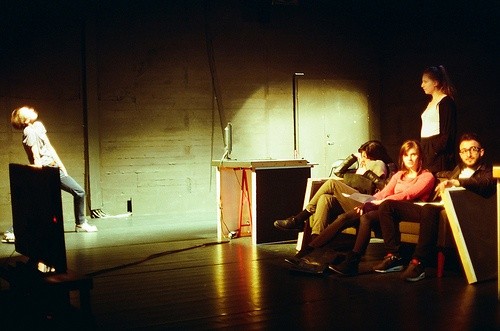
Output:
top-left (75, 222), bottom-right (98, 232)
top-left (405, 261), bottom-right (425, 281)
top-left (374, 256), bottom-right (404, 272)
top-left (2, 232), bottom-right (15, 242)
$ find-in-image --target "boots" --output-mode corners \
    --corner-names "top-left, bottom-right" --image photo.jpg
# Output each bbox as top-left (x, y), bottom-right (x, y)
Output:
top-left (329, 252), bottom-right (361, 276)
top-left (284, 245), bottom-right (314, 265)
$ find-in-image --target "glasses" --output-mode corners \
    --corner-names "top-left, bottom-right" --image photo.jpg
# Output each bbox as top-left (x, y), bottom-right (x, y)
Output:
top-left (460, 146), bottom-right (480, 155)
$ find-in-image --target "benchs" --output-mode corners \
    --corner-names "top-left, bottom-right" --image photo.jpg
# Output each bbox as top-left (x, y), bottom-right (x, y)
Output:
top-left (301, 171), bottom-right (457, 278)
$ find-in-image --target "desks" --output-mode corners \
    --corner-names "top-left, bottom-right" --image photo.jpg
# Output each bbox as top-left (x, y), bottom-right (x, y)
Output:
top-left (211, 158), bottom-right (318, 244)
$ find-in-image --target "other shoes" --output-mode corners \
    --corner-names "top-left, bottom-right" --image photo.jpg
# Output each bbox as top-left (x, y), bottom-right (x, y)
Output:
top-left (274, 216), bottom-right (304, 232)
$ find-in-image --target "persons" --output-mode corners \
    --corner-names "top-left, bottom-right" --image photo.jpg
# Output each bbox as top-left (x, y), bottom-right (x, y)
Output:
top-left (274, 141), bottom-right (398, 266)
top-left (420, 65), bottom-right (460, 176)
top-left (373, 134), bottom-right (497, 282)
top-left (285, 139), bottom-right (435, 277)
top-left (11, 105), bottom-right (98, 232)
